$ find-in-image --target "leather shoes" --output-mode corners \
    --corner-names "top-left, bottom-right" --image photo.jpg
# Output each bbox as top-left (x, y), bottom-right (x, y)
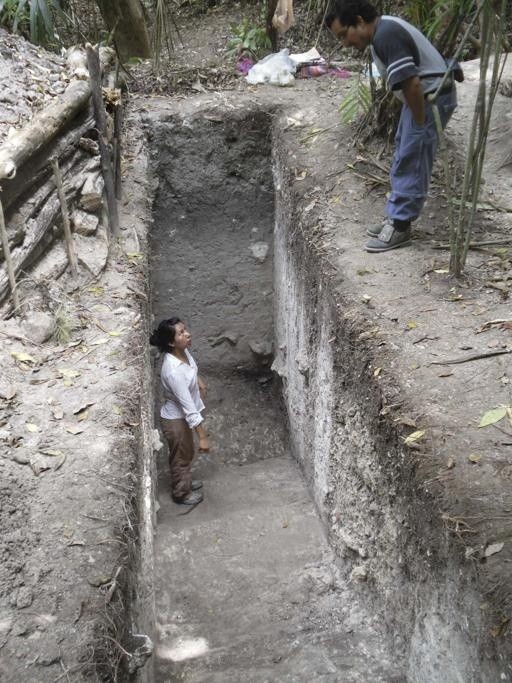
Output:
top-left (364, 216), bottom-right (411, 251)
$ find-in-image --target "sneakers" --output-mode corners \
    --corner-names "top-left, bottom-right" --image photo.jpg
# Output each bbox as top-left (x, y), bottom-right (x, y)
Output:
top-left (174, 479), bottom-right (202, 505)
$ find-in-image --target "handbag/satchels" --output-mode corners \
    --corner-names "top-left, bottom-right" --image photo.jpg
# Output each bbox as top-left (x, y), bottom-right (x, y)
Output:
top-left (446, 57), bottom-right (463, 82)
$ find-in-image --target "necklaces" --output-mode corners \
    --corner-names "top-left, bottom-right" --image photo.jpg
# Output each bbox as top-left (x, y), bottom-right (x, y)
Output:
top-left (174, 350), bottom-right (190, 365)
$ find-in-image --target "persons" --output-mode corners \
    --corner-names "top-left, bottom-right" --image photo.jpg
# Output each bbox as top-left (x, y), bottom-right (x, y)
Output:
top-left (323, 0), bottom-right (458, 252)
top-left (150, 316), bottom-right (210, 504)
